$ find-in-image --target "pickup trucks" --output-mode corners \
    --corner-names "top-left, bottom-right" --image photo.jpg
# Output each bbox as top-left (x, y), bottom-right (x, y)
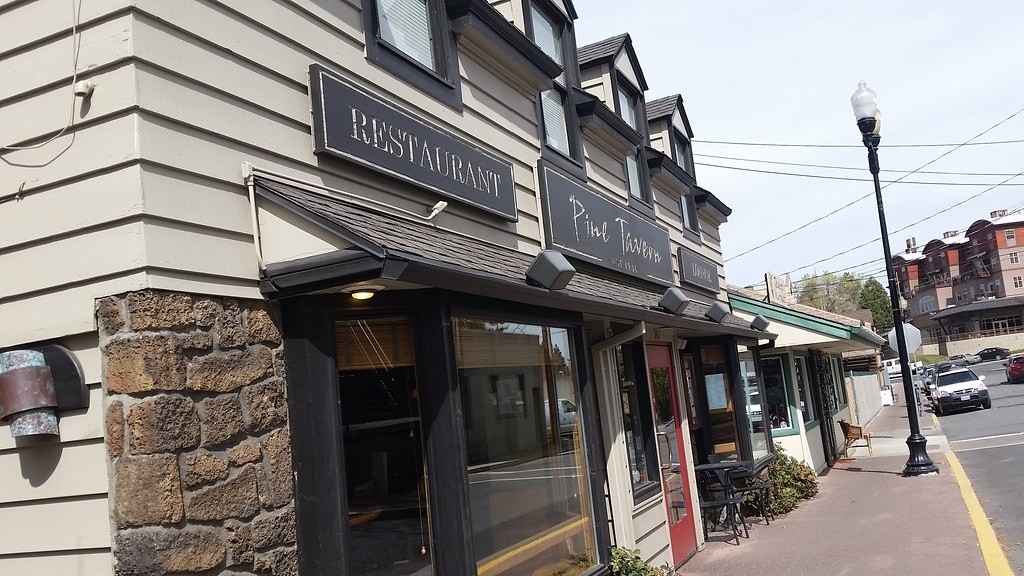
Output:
top-left (881, 357), bottom-right (923, 375)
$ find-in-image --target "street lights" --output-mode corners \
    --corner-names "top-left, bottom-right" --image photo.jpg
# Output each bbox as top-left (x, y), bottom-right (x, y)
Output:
top-left (851, 78), bottom-right (940, 477)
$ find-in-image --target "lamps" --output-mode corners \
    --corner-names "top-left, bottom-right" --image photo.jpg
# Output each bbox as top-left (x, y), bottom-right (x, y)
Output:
top-left (704, 300), bottom-right (731, 324)
top-left (657, 286), bottom-right (688, 315)
top-left (749, 314), bottom-right (770, 332)
top-left (524, 249), bottom-right (576, 290)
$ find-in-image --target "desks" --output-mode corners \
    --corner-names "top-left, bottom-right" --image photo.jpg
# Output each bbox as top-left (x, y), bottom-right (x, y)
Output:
top-left (699, 451), bottom-right (777, 527)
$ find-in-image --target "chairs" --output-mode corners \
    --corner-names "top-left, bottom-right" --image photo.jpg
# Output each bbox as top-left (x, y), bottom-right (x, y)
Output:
top-left (672, 465), bottom-right (775, 545)
top-left (837, 420), bottom-right (874, 459)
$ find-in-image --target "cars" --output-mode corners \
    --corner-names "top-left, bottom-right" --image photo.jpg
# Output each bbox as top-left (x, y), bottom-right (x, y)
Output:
top-left (1002, 354), bottom-right (1024, 384)
top-left (929, 368), bottom-right (991, 416)
top-left (946, 353), bottom-right (983, 366)
top-left (917, 362), bottom-right (960, 396)
top-left (974, 347), bottom-right (1011, 361)
